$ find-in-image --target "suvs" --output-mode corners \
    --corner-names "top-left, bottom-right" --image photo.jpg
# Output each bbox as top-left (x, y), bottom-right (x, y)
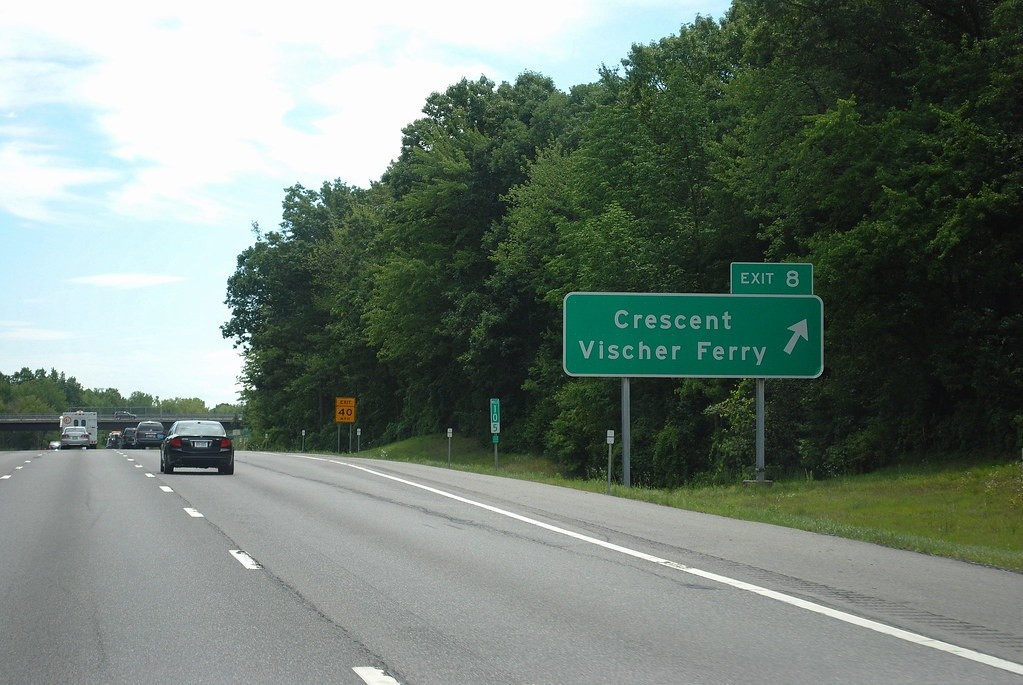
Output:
top-left (105, 427), bottom-right (145, 449)
top-left (114, 411), bottom-right (137, 420)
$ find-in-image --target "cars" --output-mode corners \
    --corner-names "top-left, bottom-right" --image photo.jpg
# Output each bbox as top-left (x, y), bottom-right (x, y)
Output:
top-left (59, 426), bottom-right (92, 449)
top-left (49, 440), bottom-right (61, 450)
top-left (133, 421), bottom-right (167, 448)
top-left (157, 420), bottom-right (235, 475)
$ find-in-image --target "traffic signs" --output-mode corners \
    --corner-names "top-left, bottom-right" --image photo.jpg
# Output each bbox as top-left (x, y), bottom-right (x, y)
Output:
top-left (562, 262), bottom-right (825, 380)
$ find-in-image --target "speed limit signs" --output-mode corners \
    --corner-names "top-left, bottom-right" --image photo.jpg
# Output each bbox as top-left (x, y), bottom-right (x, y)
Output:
top-left (335, 397), bottom-right (355, 423)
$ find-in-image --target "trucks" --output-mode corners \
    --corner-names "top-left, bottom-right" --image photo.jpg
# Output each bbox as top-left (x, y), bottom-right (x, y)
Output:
top-left (59, 411), bottom-right (97, 450)
